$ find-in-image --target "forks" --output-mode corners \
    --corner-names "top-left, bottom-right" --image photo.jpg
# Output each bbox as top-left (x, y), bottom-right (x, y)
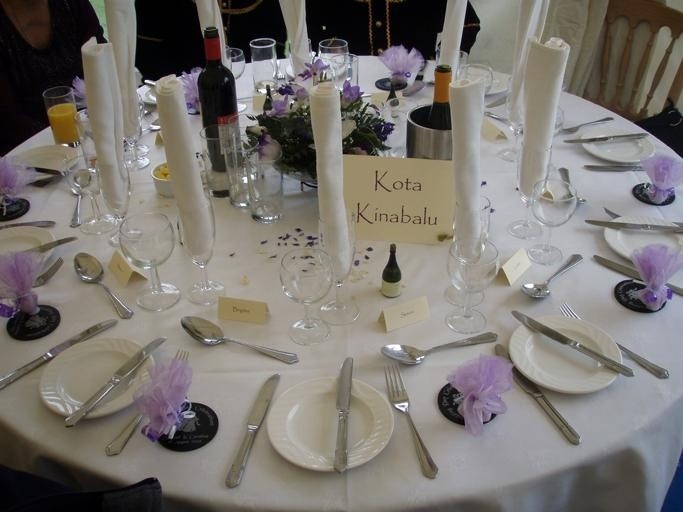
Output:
top-left (385, 365), bottom-right (443, 481)
top-left (1, 257), bottom-right (65, 299)
top-left (559, 117), bottom-right (614, 135)
top-left (559, 302), bottom-right (671, 379)
top-left (103, 349), bottom-right (191, 456)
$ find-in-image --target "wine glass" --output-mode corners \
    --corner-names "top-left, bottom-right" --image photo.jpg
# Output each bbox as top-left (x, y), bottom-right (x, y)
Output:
top-left (509, 142), bottom-right (553, 240)
top-left (284, 38), bottom-right (314, 79)
top-left (120, 212), bottom-right (181, 312)
top-left (330, 51), bottom-right (360, 90)
top-left (250, 37), bottom-right (279, 96)
top-left (280, 247), bottom-right (330, 345)
top-left (526, 179), bottom-right (579, 265)
top-left (495, 76), bottom-right (526, 162)
top-left (317, 212), bottom-right (360, 323)
top-left (445, 238), bottom-right (500, 333)
top-left (443, 197), bottom-right (491, 307)
top-left (318, 38), bottom-right (349, 79)
top-left (225, 48), bottom-right (248, 115)
top-left (66, 154), bottom-right (120, 236)
top-left (457, 62), bottom-right (495, 96)
top-left (174, 189), bottom-right (226, 305)
top-left (386, 97), bottom-right (418, 159)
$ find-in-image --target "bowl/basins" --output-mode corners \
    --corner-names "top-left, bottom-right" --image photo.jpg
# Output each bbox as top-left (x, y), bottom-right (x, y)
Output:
top-left (151, 160), bottom-right (174, 198)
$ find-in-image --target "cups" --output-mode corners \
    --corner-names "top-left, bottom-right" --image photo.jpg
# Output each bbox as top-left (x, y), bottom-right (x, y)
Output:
top-left (42, 86), bottom-right (82, 150)
top-left (200, 125), bottom-right (234, 198)
top-left (223, 135), bottom-right (260, 208)
top-left (246, 147), bottom-right (285, 224)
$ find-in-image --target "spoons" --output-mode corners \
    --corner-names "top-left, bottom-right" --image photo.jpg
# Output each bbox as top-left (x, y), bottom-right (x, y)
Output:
top-left (520, 253), bottom-right (583, 299)
top-left (180, 314), bottom-right (299, 367)
top-left (74, 252), bottom-right (134, 321)
top-left (67, 163), bottom-right (97, 226)
top-left (381, 331), bottom-right (498, 365)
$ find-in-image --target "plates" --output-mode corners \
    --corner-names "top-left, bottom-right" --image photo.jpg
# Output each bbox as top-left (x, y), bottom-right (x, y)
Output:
top-left (38, 339), bottom-right (155, 419)
top-left (266, 375), bottom-right (394, 471)
top-left (604, 214), bottom-right (681, 262)
top-left (581, 130), bottom-right (657, 162)
top-left (506, 315), bottom-right (625, 394)
top-left (136, 79), bottom-right (159, 108)
top-left (16, 144), bottom-right (80, 185)
top-left (1, 226), bottom-right (58, 257)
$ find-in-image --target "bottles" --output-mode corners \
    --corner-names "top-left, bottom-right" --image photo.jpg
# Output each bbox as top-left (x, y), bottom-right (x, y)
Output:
top-left (196, 27), bottom-right (244, 173)
top-left (380, 244), bottom-right (403, 299)
top-left (427, 62), bottom-right (452, 131)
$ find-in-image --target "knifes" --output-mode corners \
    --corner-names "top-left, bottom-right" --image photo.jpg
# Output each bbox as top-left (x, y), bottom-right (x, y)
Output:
top-left (585, 218), bottom-right (681, 234)
top-left (63, 335), bottom-right (168, 429)
top-left (2, 237), bottom-right (78, 255)
top-left (0, 319), bottom-right (118, 390)
top-left (331, 355), bottom-right (352, 472)
top-left (594, 256), bottom-right (682, 296)
top-left (222, 372), bottom-right (278, 487)
top-left (494, 341), bottom-right (585, 448)
top-left (563, 132), bottom-right (650, 143)
top-left (584, 164), bottom-right (647, 173)
top-left (511, 310), bottom-right (635, 378)
